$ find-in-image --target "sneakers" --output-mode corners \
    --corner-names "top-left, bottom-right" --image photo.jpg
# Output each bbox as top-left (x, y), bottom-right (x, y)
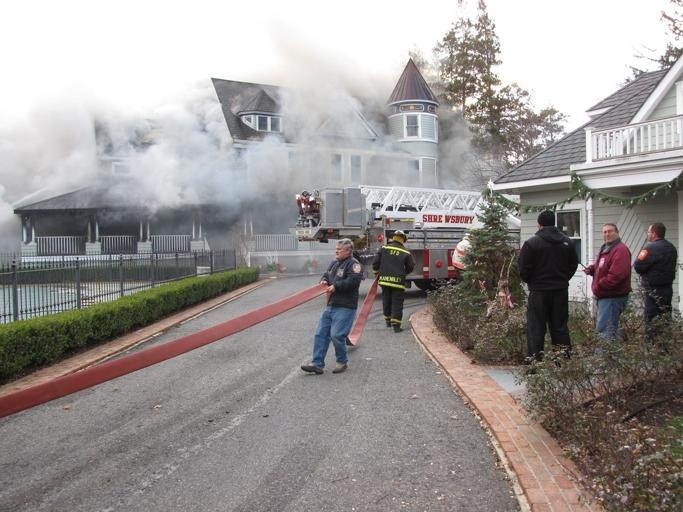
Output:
top-left (299, 363), bottom-right (324, 374)
top-left (331, 361), bottom-right (347, 373)
top-left (385, 320), bottom-right (404, 332)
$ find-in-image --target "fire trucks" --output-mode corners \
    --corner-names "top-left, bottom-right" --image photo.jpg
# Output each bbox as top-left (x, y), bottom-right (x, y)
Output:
top-left (294, 183), bottom-right (522, 290)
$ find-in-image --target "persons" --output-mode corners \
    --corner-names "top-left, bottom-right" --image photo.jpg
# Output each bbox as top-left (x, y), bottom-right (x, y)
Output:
top-left (582, 221), bottom-right (631, 375)
top-left (451, 232), bottom-right (474, 281)
top-left (372, 229), bottom-right (416, 334)
top-left (301, 239), bottom-right (363, 374)
top-left (635, 221), bottom-right (679, 356)
top-left (518, 211), bottom-right (579, 377)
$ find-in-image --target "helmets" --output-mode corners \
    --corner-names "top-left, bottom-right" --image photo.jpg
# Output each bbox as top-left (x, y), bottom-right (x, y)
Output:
top-left (392, 229), bottom-right (407, 243)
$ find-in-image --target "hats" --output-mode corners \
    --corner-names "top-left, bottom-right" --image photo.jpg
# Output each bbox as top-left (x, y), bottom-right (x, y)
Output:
top-left (536, 209), bottom-right (556, 226)
